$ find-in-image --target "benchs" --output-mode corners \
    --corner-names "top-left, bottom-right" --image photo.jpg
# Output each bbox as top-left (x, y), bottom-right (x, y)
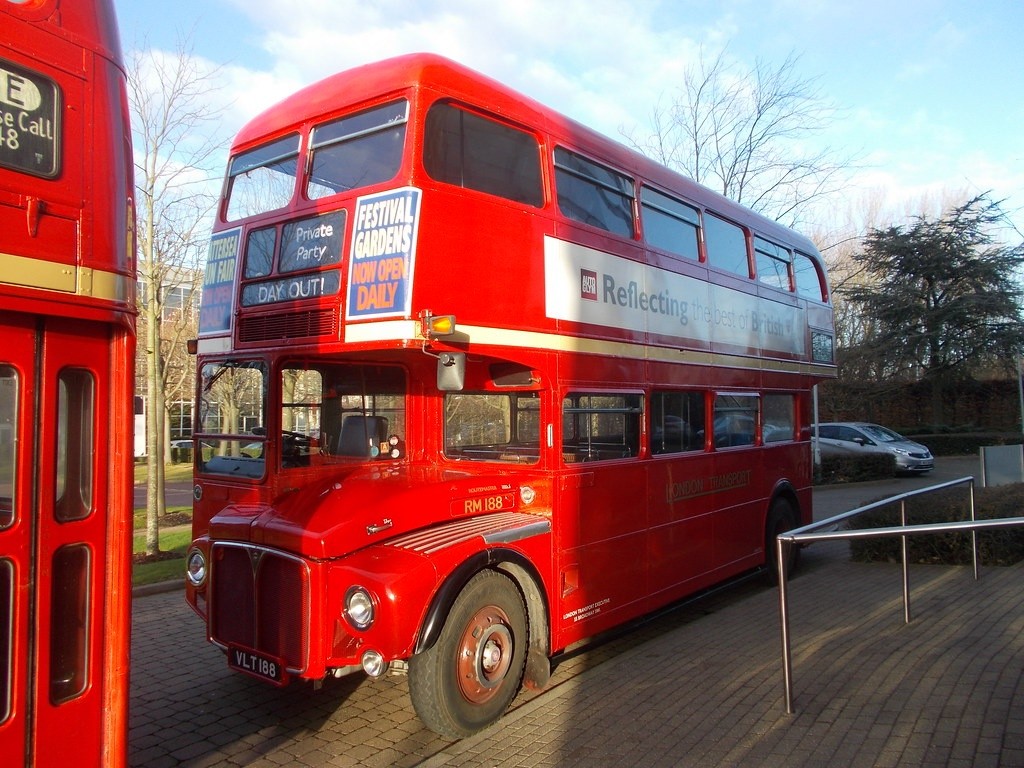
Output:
top-left (500, 441), bottom-right (660, 462)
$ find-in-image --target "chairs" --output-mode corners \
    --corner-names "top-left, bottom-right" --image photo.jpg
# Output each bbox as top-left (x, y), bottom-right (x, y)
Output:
top-left (337, 415), bottom-right (388, 456)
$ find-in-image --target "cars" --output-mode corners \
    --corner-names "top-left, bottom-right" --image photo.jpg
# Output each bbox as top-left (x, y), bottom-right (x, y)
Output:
top-left (695, 413), bottom-right (783, 447)
top-left (170, 439), bottom-right (211, 449)
top-left (658, 415), bottom-right (693, 435)
top-left (811, 421), bottom-right (936, 475)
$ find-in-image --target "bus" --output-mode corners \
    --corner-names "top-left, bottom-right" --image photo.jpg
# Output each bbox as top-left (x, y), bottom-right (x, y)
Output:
top-left (181, 53), bottom-right (840, 740)
top-left (0, 0), bottom-right (140, 767)
